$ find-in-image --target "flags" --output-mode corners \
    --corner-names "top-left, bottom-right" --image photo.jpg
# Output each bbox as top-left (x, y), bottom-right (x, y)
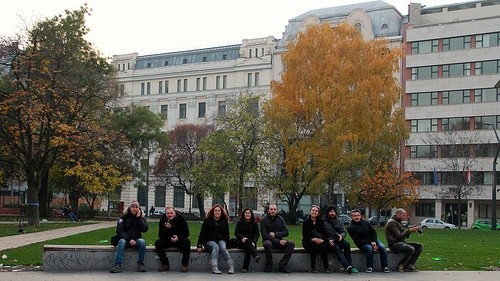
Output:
top-left (433, 167), bottom-right (438, 186)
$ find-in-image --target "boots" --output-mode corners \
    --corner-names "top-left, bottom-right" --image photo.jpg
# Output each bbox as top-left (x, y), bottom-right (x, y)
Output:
top-left (226, 259), bottom-right (235, 273)
top-left (209, 258), bottom-right (222, 274)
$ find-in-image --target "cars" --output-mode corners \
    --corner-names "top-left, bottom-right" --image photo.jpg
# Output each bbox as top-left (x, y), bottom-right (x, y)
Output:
top-left (420, 217), bottom-right (456, 230)
top-left (471, 218), bottom-right (500, 231)
top-left (368, 215), bottom-right (387, 226)
top-left (339, 215), bottom-right (353, 224)
top-left (401, 220), bottom-right (407, 227)
top-left (154, 210), bottom-right (165, 216)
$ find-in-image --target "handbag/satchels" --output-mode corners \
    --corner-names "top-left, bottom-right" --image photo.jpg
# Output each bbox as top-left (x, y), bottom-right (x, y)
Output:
top-left (110, 235), bottom-right (121, 247)
top-left (231, 238), bottom-right (238, 248)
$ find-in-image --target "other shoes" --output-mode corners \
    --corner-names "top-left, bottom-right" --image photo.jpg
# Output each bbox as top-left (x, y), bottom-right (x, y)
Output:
top-left (254, 256), bottom-right (261, 263)
top-left (405, 264), bottom-right (419, 272)
top-left (241, 269), bottom-right (247, 272)
top-left (311, 268), bottom-right (318, 273)
top-left (264, 265), bottom-right (273, 272)
top-left (397, 265), bottom-right (404, 272)
top-left (279, 267), bottom-right (291, 273)
top-left (109, 265), bottom-right (122, 273)
top-left (158, 265), bottom-right (169, 272)
top-left (136, 263), bottom-right (147, 272)
top-left (365, 267), bottom-right (372, 273)
top-left (383, 266), bottom-right (391, 273)
top-left (182, 266), bottom-right (188, 273)
top-left (346, 266), bottom-right (353, 273)
top-left (324, 269), bottom-right (330, 273)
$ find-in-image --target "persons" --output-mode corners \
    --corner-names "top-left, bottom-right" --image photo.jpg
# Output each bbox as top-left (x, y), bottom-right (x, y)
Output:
top-left (301, 204), bottom-right (332, 274)
top-left (197, 204), bottom-right (235, 274)
top-left (109, 201), bottom-right (149, 272)
top-left (385, 208), bottom-right (424, 272)
top-left (260, 204), bottom-right (295, 273)
top-left (154, 207), bottom-right (192, 272)
top-left (234, 208), bottom-right (260, 272)
top-left (63, 205), bottom-right (80, 222)
top-left (348, 209), bottom-right (391, 273)
top-left (150, 205), bottom-right (155, 216)
top-left (322, 206), bottom-right (359, 273)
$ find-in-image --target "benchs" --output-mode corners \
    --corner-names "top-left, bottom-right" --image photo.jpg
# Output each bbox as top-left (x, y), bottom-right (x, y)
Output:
top-left (52, 209), bottom-right (67, 222)
top-left (0, 208), bottom-right (25, 220)
top-left (44, 243), bottom-right (404, 271)
